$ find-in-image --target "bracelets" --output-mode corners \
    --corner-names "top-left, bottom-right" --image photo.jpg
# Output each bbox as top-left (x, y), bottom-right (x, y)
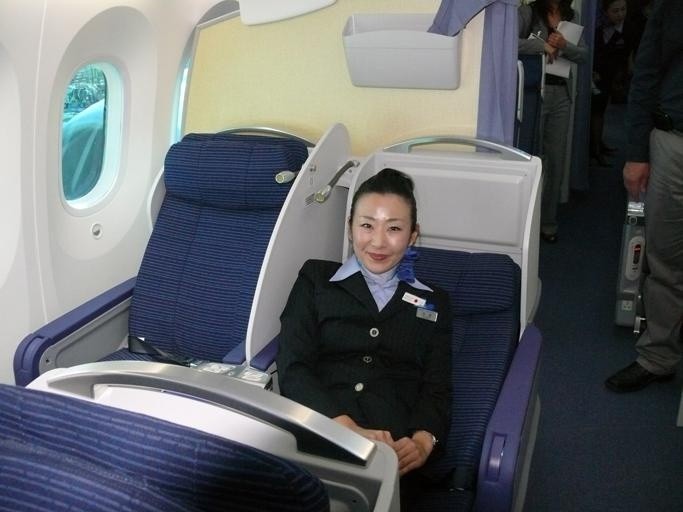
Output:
top-left (423, 430), bottom-right (438, 445)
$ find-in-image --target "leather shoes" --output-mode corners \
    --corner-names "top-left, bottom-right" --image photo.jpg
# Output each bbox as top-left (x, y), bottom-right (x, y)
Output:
top-left (604, 354), bottom-right (677, 395)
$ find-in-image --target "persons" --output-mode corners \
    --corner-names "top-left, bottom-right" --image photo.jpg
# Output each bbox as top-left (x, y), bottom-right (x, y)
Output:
top-left (518, 0), bottom-right (589, 244)
top-left (593, 0), bottom-right (683, 393)
top-left (277, 168), bottom-right (452, 512)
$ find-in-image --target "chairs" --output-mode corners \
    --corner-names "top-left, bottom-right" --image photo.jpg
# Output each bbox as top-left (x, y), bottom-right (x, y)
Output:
top-left (0, 358), bottom-right (405, 510)
top-left (249, 123), bottom-right (546, 511)
top-left (515, 51), bottom-right (546, 157)
top-left (2, 124), bottom-right (320, 412)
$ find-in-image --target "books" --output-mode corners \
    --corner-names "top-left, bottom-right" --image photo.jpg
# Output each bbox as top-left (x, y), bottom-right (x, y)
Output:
top-left (545, 21), bottom-right (585, 79)
